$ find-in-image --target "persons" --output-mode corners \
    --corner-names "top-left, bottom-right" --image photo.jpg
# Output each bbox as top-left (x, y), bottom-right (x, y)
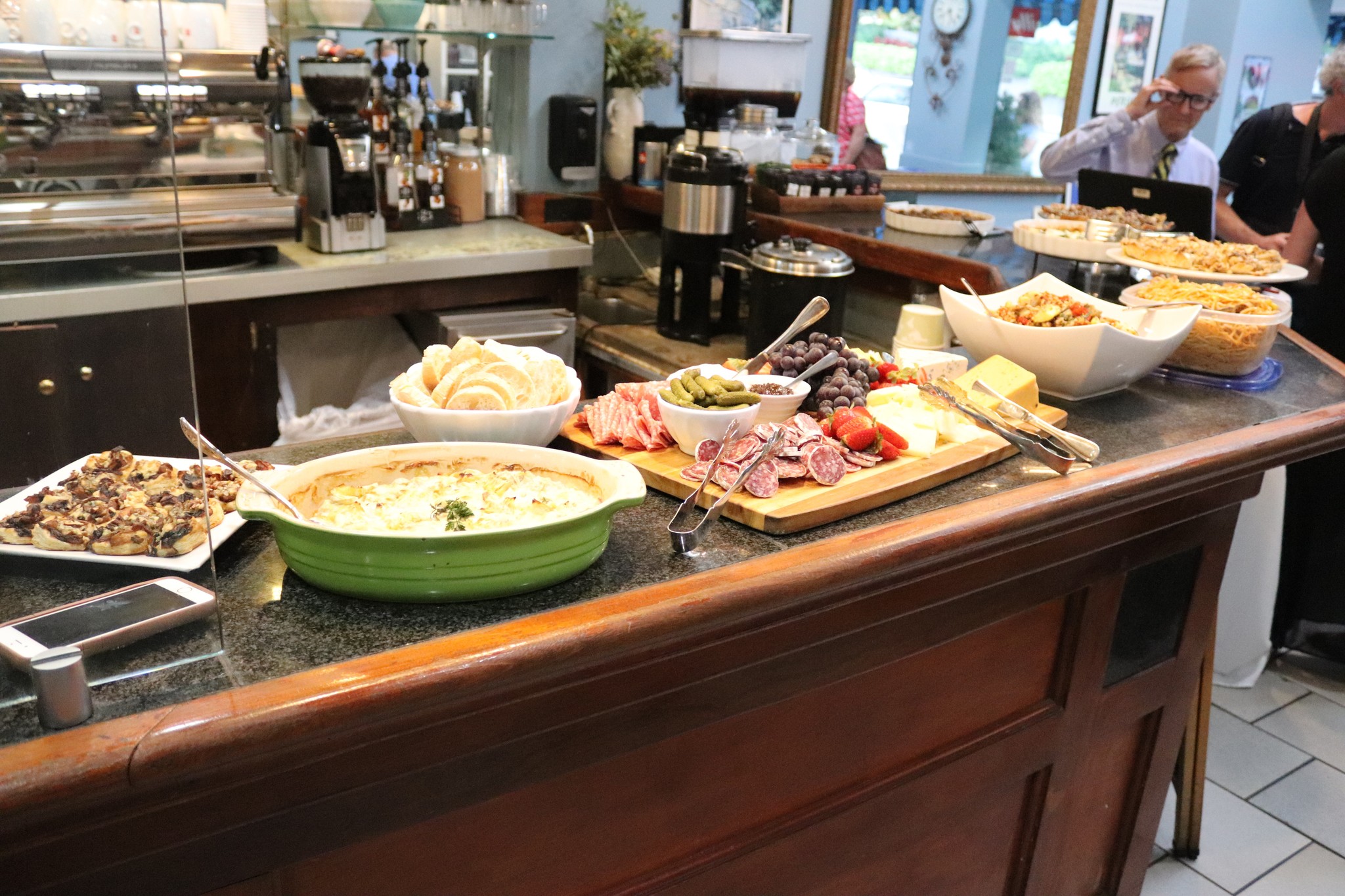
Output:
top-left (1211, 45), bottom-right (1345, 688)
top-left (1010, 92), bottom-right (1042, 177)
top-left (837, 57), bottom-right (888, 171)
top-left (370, 40), bottom-right (438, 129)
top-left (1040, 44), bottom-right (1227, 242)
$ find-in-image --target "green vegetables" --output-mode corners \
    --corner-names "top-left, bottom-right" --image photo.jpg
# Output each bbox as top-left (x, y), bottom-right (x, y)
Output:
top-left (431, 498), bottom-right (472, 533)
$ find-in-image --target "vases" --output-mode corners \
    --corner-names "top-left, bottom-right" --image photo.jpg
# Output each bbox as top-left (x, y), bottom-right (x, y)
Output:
top-left (604, 87), bottom-right (643, 182)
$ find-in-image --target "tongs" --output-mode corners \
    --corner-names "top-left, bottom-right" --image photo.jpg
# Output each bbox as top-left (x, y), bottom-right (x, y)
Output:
top-left (666, 418), bottom-right (788, 553)
top-left (917, 375), bottom-right (1076, 476)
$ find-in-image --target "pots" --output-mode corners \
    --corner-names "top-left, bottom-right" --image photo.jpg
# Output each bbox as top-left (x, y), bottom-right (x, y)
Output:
top-left (747, 231), bottom-right (856, 356)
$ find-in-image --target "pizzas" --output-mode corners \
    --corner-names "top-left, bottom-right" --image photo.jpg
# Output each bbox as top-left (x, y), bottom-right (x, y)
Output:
top-left (1041, 203), bottom-right (1167, 230)
top-left (1121, 233), bottom-right (1289, 277)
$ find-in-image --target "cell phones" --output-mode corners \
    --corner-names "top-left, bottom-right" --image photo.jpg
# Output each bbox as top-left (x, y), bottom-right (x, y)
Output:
top-left (0, 575), bottom-right (215, 675)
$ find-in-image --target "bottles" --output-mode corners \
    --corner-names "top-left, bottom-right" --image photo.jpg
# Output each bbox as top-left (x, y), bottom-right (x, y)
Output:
top-left (726, 104), bottom-right (842, 203)
top-left (365, 76), bottom-right (498, 230)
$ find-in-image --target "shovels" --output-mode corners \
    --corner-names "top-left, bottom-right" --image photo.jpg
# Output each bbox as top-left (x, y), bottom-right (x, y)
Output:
top-left (961, 218), bottom-right (1005, 238)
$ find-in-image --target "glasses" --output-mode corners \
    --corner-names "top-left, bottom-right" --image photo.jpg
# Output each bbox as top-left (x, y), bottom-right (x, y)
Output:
top-left (1165, 88), bottom-right (1215, 112)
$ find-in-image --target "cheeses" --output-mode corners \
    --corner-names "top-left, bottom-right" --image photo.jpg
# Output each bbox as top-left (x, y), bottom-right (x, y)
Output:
top-left (862, 345), bottom-right (1038, 456)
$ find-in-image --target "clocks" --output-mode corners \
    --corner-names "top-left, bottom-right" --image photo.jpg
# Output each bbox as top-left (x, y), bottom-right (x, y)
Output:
top-left (925, 0), bottom-right (971, 112)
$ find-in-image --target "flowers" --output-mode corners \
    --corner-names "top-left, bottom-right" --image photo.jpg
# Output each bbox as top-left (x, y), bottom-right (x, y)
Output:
top-left (593, 4), bottom-right (679, 91)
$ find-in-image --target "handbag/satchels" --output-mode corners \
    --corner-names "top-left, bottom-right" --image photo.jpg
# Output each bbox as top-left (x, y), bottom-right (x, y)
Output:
top-left (854, 138), bottom-right (886, 171)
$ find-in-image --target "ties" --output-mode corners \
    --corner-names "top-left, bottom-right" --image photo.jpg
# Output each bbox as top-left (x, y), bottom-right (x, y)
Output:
top-left (1149, 142), bottom-right (1178, 181)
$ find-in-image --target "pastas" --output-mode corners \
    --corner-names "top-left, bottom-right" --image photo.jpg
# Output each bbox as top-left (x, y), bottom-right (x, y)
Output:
top-left (1137, 277), bottom-right (1280, 376)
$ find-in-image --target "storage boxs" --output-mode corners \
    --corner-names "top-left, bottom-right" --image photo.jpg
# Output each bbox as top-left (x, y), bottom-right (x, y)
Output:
top-left (1118, 281), bottom-right (1294, 376)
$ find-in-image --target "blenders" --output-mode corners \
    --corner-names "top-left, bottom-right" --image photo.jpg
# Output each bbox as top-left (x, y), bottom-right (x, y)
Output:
top-left (292, 56), bottom-right (386, 256)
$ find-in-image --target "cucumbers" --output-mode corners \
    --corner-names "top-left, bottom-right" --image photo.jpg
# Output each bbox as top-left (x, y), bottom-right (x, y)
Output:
top-left (658, 367), bottom-right (762, 411)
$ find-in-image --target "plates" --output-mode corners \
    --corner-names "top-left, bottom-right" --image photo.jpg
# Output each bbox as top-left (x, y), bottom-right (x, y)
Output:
top-left (1105, 248), bottom-right (1309, 284)
top-left (0, 452), bottom-right (296, 573)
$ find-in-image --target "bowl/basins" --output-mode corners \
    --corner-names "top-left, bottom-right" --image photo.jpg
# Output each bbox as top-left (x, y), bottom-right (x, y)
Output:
top-left (234, 442), bottom-right (648, 603)
top-left (731, 374), bottom-right (812, 426)
top-left (936, 271), bottom-right (1204, 402)
top-left (1010, 218), bottom-right (1116, 264)
top-left (1118, 278), bottom-right (1294, 377)
top-left (883, 202), bottom-right (996, 236)
top-left (656, 388), bottom-right (761, 457)
top-left (388, 359), bottom-right (583, 448)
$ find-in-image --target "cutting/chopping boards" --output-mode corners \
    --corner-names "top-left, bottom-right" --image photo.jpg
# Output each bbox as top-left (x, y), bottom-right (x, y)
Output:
top-left (558, 404), bottom-right (1069, 536)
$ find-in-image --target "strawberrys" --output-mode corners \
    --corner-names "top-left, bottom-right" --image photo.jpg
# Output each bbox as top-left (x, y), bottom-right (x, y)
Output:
top-left (820, 358), bottom-right (926, 461)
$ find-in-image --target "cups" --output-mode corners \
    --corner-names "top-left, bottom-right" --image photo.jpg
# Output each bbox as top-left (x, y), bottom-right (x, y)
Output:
top-left (416, 0), bottom-right (547, 37)
top-left (889, 302), bottom-right (947, 358)
top-left (0, 0), bottom-right (270, 51)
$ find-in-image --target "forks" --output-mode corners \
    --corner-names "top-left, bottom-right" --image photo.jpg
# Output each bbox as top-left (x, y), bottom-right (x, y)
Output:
top-left (962, 219), bottom-right (1008, 238)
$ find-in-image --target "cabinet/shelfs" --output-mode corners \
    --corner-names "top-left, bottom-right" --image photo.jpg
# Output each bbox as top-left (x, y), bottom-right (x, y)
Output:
top-left (0, 301), bottom-right (255, 489)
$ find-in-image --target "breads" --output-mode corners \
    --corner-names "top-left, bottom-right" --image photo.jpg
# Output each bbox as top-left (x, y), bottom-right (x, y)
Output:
top-left (391, 338), bottom-right (570, 411)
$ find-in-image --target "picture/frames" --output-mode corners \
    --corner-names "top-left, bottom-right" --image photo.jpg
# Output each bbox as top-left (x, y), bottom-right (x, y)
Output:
top-left (683, 0), bottom-right (791, 32)
top-left (1092, 0), bottom-right (1167, 117)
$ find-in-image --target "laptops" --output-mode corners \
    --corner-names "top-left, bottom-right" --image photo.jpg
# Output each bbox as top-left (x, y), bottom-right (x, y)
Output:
top-left (1076, 167), bottom-right (1212, 242)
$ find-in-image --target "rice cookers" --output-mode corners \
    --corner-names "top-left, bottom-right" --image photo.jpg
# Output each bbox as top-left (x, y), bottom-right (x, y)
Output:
top-left (660, 151), bottom-right (751, 245)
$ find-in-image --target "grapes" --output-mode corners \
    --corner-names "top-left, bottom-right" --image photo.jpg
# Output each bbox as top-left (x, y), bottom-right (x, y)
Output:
top-left (769, 331), bottom-right (880, 419)
